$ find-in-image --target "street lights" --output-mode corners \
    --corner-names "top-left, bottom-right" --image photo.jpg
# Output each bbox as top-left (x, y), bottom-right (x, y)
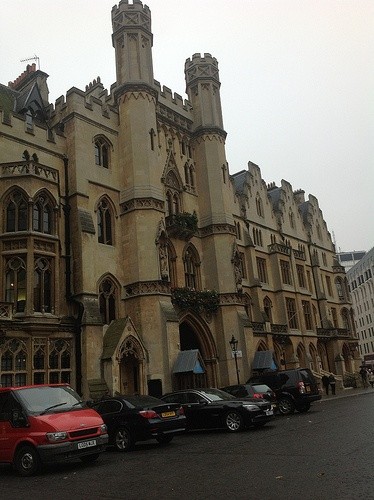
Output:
top-left (230, 334), bottom-right (242, 382)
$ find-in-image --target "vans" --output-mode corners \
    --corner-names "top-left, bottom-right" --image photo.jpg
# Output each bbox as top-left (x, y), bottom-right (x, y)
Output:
top-left (1, 383), bottom-right (109, 472)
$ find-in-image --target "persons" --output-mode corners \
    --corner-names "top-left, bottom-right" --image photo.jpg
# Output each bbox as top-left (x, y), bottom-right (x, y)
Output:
top-left (329, 372), bottom-right (336, 395)
top-left (359, 368), bottom-right (374, 388)
top-left (321, 373), bottom-right (329, 396)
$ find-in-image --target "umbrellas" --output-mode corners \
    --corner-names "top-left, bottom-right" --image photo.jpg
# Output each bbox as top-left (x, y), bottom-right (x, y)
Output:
top-left (359, 364), bottom-right (369, 368)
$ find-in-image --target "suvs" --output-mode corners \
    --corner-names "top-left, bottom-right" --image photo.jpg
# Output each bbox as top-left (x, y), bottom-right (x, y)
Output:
top-left (239, 368), bottom-right (322, 416)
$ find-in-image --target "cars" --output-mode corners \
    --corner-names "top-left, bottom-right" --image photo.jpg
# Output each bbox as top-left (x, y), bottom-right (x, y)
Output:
top-left (221, 383), bottom-right (287, 418)
top-left (87, 393), bottom-right (186, 452)
top-left (160, 388), bottom-right (277, 431)
